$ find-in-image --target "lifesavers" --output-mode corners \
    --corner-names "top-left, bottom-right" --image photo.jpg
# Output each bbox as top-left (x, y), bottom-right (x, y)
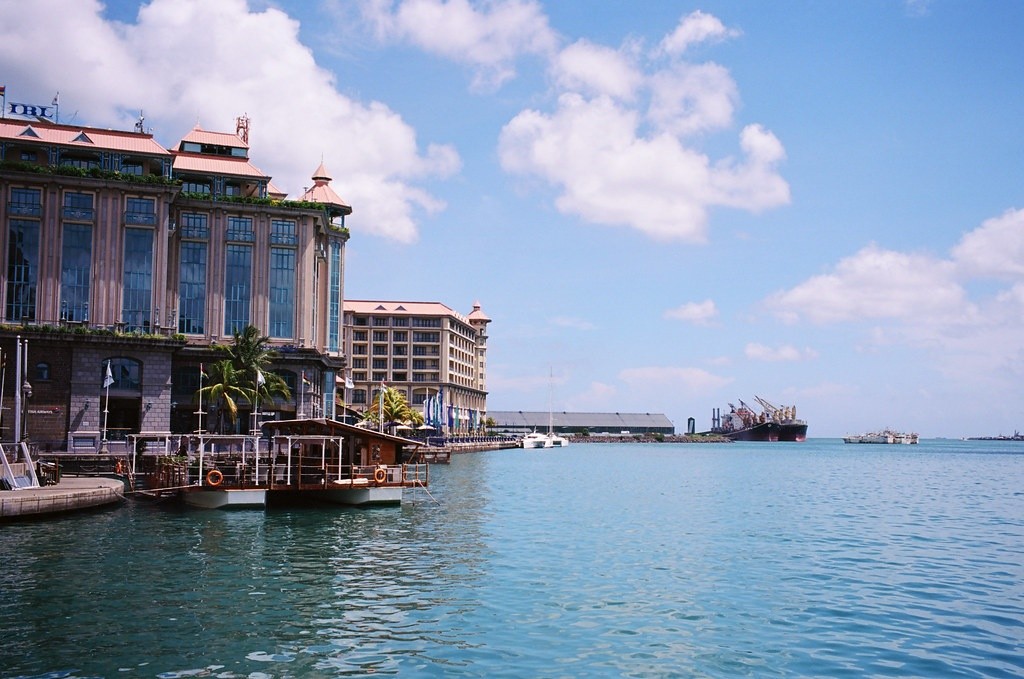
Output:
top-left (207, 470), bottom-right (223, 486)
top-left (374, 468), bottom-right (386, 484)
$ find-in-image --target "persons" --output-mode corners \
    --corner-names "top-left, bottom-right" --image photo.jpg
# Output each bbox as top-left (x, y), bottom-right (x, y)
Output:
top-left (175, 446), bottom-right (188, 457)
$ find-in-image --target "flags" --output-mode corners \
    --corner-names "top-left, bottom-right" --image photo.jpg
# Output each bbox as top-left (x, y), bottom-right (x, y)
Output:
top-left (258, 371), bottom-right (265, 384)
top-left (304, 374), bottom-right (311, 385)
top-left (103, 363), bottom-right (114, 388)
top-left (346, 377), bottom-right (354, 389)
top-left (202, 368), bottom-right (209, 379)
top-left (0, 86), bottom-right (4, 96)
top-left (383, 381), bottom-right (387, 393)
top-left (52, 95), bottom-right (58, 105)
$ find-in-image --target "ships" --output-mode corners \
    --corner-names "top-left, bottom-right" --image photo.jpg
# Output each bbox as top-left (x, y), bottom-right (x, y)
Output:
top-left (711, 394), bottom-right (807, 442)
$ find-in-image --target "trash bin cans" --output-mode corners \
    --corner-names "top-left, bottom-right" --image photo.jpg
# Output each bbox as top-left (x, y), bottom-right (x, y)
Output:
top-left (51, 464), bottom-right (64, 481)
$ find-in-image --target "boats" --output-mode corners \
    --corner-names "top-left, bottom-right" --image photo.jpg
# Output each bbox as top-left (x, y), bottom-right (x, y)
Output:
top-left (257, 418), bottom-right (429, 505)
top-left (125, 433), bottom-right (269, 507)
